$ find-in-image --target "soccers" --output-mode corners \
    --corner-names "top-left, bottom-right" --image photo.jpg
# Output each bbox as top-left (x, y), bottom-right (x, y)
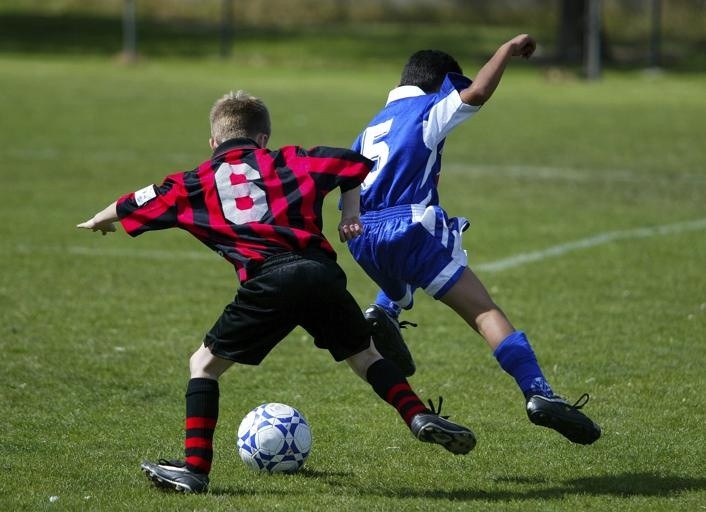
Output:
top-left (238, 403), bottom-right (311, 473)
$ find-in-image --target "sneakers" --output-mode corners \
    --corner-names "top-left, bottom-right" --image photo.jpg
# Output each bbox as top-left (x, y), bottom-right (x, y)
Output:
top-left (141, 459), bottom-right (209, 495)
top-left (364, 303), bottom-right (417, 377)
top-left (411, 396), bottom-right (476, 455)
top-left (527, 392), bottom-right (601, 445)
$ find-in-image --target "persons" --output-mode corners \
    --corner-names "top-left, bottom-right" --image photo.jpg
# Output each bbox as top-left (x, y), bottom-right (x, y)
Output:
top-left (75, 89), bottom-right (476, 494)
top-left (337, 32), bottom-right (601, 446)
